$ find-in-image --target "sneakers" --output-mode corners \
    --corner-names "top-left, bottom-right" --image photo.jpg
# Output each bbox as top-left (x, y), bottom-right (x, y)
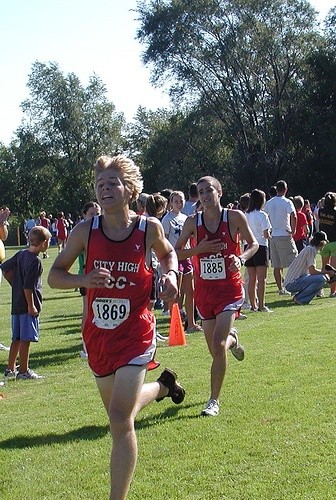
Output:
top-left (249, 306), bottom-right (273, 313)
top-left (279, 287), bottom-right (291, 295)
top-left (16, 368), bottom-right (46, 380)
top-left (155, 367), bottom-right (185, 404)
top-left (228, 329), bottom-right (245, 361)
top-left (201, 397), bottom-right (220, 416)
top-left (237, 312), bottom-right (247, 320)
top-left (3, 364), bottom-right (21, 380)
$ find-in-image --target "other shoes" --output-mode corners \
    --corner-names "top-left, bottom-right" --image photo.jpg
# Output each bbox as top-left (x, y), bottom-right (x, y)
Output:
top-left (317, 289), bottom-right (325, 298)
top-left (293, 296), bottom-right (312, 304)
top-left (43, 253), bottom-right (50, 258)
top-left (154, 300), bottom-right (203, 332)
top-left (330, 291), bottom-right (336, 297)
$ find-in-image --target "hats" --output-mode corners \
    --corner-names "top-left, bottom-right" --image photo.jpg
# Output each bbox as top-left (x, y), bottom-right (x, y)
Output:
top-left (138, 193), bottom-right (150, 204)
top-left (314, 231), bottom-right (329, 243)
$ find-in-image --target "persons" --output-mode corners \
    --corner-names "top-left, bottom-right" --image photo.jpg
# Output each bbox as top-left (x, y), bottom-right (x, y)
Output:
top-left (0, 225), bottom-right (52, 380)
top-left (136, 184), bottom-right (203, 331)
top-left (47, 154), bottom-right (185, 500)
top-left (74, 202), bottom-right (101, 328)
top-left (175, 176), bottom-right (259, 415)
top-left (284, 231), bottom-right (335, 305)
top-left (316, 192), bottom-right (336, 296)
top-left (25, 211), bottom-right (73, 258)
top-left (264, 180), bottom-right (298, 296)
top-left (243, 189), bottom-right (273, 313)
top-left (0, 207), bottom-right (11, 282)
top-left (227, 192), bottom-right (336, 294)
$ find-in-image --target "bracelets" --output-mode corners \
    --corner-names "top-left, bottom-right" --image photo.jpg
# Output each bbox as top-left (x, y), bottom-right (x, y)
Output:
top-left (166, 269), bottom-right (180, 280)
top-left (239, 257), bottom-right (243, 266)
